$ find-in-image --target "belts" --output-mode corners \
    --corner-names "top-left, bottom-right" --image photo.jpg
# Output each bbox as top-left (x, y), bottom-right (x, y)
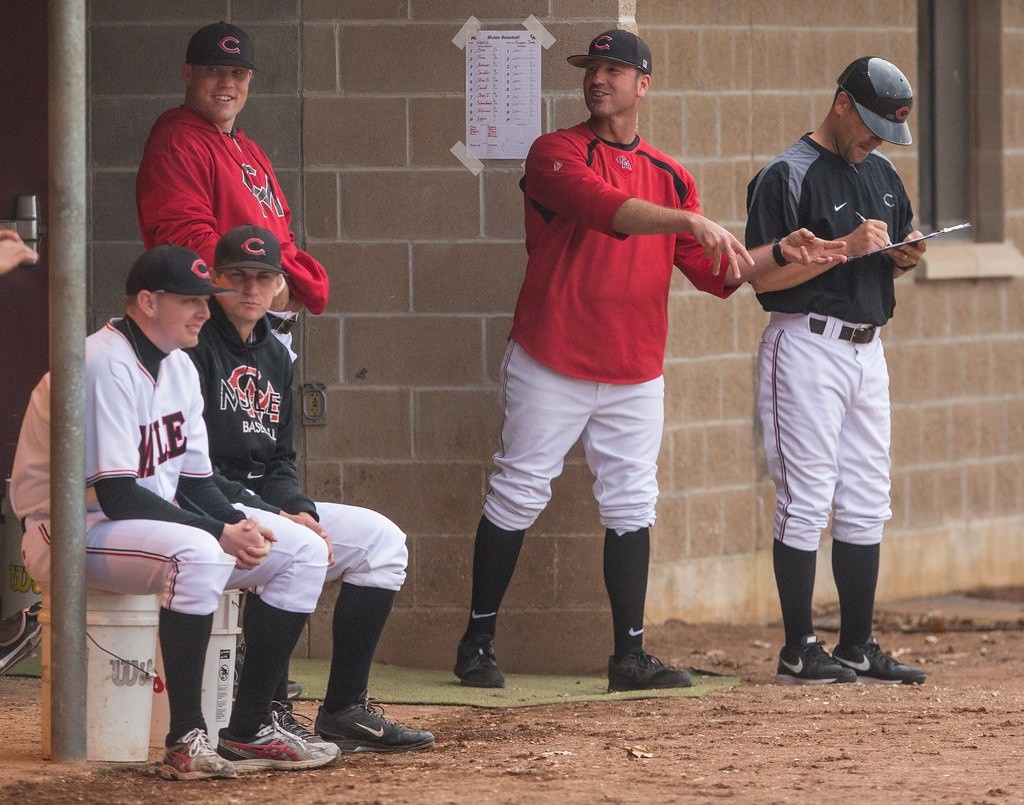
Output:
top-left (809, 316), bottom-right (876, 344)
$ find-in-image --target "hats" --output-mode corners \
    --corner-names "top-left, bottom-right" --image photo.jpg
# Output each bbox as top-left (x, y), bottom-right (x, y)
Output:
top-left (125, 245), bottom-right (236, 294)
top-left (567, 29), bottom-right (653, 75)
top-left (215, 224), bottom-right (289, 277)
top-left (185, 21), bottom-right (255, 70)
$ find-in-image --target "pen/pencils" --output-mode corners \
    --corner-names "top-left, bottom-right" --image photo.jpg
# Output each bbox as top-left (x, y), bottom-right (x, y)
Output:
top-left (854, 211), bottom-right (892, 246)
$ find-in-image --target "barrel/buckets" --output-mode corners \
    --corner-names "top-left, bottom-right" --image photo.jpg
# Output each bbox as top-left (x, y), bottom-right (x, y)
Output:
top-left (37, 587), bottom-right (242, 763)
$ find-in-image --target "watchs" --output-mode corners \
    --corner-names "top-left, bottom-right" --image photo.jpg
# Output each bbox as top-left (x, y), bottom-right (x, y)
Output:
top-left (894, 261), bottom-right (917, 272)
top-left (773, 240), bottom-right (792, 267)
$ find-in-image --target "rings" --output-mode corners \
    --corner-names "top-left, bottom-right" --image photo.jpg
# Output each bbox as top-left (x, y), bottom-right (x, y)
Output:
top-left (902, 255), bottom-right (907, 260)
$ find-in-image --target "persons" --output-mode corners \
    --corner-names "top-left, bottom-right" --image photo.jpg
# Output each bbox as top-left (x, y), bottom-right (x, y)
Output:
top-left (745, 56), bottom-right (927, 687)
top-left (452, 30), bottom-right (846, 694)
top-left (136, 21), bottom-right (329, 363)
top-left (1, 228), bottom-right (38, 275)
top-left (180, 222), bottom-right (435, 753)
top-left (9, 241), bottom-right (340, 780)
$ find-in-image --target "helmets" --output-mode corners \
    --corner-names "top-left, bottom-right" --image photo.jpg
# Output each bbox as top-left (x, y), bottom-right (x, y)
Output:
top-left (836, 55), bottom-right (913, 147)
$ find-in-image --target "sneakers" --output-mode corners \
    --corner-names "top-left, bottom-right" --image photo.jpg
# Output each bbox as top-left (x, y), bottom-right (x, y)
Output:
top-left (453, 633), bottom-right (505, 687)
top-left (831, 632), bottom-right (926, 684)
top-left (157, 727), bottom-right (237, 779)
top-left (233, 645), bottom-right (301, 698)
top-left (312, 690), bottom-right (435, 753)
top-left (607, 654), bottom-right (691, 691)
top-left (776, 634), bottom-right (857, 686)
top-left (216, 711), bottom-right (338, 772)
top-left (274, 709), bottom-right (325, 743)
